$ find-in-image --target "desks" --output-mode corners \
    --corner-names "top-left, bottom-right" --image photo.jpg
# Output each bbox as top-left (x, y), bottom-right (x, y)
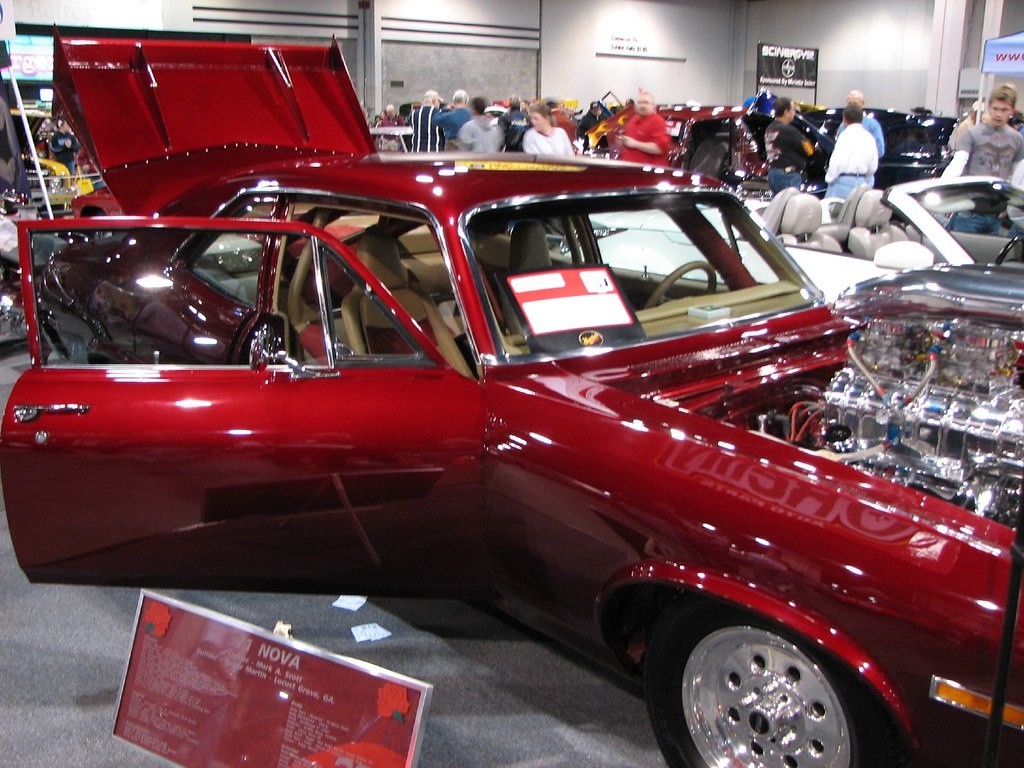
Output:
top-left (369, 125), bottom-right (414, 154)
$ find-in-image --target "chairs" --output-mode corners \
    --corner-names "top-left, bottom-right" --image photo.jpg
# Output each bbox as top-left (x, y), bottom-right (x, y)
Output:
top-left (689, 138), bottom-right (730, 178)
top-left (493, 223), bottom-right (556, 334)
top-left (759, 182), bottom-right (911, 260)
top-left (340, 231), bottom-right (476, 381)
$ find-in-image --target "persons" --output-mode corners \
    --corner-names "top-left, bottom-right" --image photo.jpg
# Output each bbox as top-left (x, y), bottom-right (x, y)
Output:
top-left (359, 84), bottom-right (1024, 237)
top-left (49, 119), bottom-right (106, 191)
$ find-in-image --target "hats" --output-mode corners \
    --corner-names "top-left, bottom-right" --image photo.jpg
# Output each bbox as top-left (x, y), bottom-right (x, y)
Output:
top-left (452, 89), bottom-right (468, 103)
top-left (425, 89), bottom-right (443, 102)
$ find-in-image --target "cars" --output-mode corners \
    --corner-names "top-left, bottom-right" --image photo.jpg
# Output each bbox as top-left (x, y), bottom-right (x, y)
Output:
top-left (656, 104), bottom-right (769, 182)
top-left (5, 109), bottom-right (94, 216)
top-left (744, 104), bottom-right (957, 188)
top-left (0, 20), bottom-right (1024, 768)
